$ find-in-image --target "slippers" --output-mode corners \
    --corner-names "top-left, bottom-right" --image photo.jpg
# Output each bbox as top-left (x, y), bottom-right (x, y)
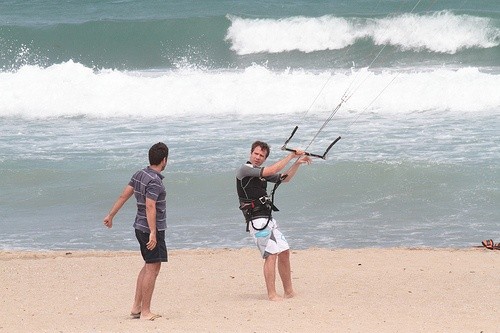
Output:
top-left (130, 311), bottom-right (141, 319)
top-left (146, 314), bottom-right (169, 321)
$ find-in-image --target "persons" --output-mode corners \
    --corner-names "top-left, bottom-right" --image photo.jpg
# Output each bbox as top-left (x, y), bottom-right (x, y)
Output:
top-left (236, 141), bottom-right (312, 301)
top-left (104, 142), bottom-right (170, 321)
